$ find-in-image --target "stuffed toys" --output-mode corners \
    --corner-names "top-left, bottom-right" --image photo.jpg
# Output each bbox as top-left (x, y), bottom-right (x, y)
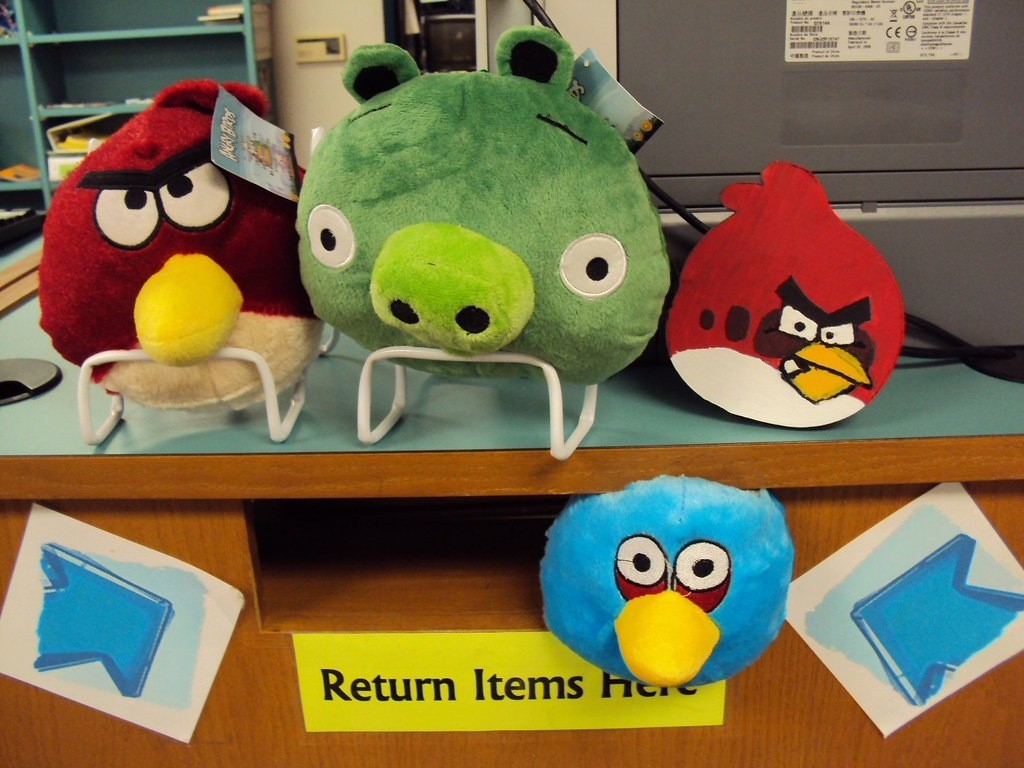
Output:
top-left (37, 79), bottom-right (326, 414)
top-left (295, 26), bottom-right (669, 389)
top-left (539, 475), bottom-right (794, 691)
top-left (669, 162), bottom-right (906, 429)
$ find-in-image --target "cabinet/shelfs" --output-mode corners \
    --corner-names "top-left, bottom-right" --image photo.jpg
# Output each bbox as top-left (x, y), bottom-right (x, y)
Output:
top-left (0, 0), bottom-right (273, 220)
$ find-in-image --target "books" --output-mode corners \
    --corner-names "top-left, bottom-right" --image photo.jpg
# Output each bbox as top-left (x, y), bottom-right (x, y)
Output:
top-left (0, 163), bottom-right (42, 182)
top-left (198, 4), bottom-right (244, 26)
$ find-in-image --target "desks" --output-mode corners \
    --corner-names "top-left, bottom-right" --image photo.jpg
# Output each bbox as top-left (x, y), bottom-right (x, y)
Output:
top-left (0, 207), bottom-right (1024, 768)
